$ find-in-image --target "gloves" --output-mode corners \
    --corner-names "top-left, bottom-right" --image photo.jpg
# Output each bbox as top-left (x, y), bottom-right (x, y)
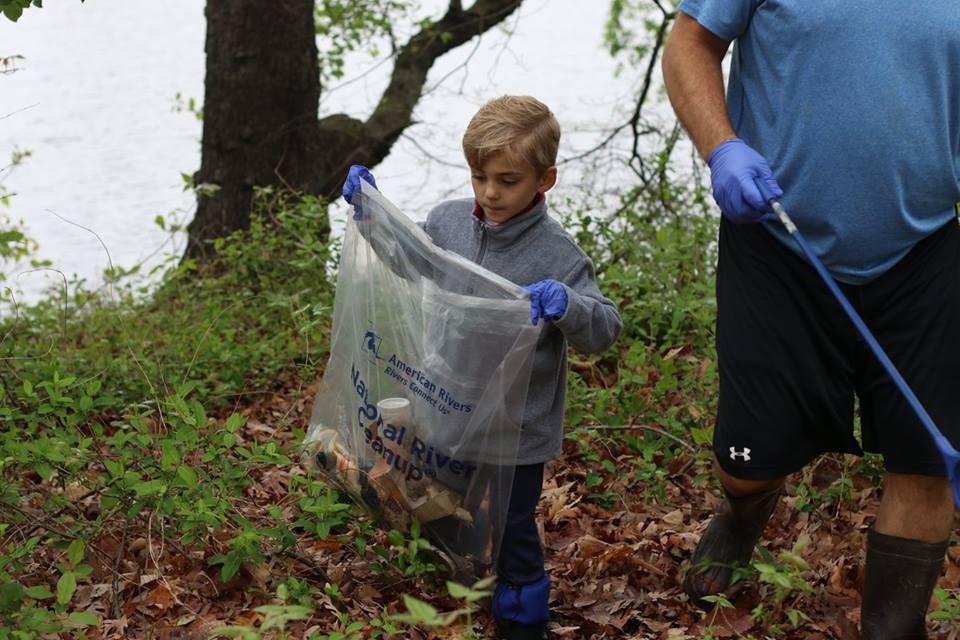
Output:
top-left (706, 138), bottom-right (786, 227)
top-left (523, 279), bottom-right (569, 328)
top-left (341, 164), bottom-right (380, 221)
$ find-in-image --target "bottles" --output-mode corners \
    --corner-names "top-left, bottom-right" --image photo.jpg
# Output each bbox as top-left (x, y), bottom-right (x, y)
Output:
top-left (377, 397), bottom-right (414, 468)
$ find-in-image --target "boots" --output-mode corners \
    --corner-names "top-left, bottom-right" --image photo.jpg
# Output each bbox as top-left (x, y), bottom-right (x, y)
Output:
top-left (488, 575), bottom-right (551, 640)
top-left (684, 480), bottom-right (784, 611)
top-left (861, 520), bottom-right (950, 640)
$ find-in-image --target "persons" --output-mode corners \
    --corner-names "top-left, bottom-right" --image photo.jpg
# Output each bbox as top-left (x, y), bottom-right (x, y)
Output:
top-left (662, 0), bottom-right (960, 638)
top-left (341, 93), bottom-right (626, 639)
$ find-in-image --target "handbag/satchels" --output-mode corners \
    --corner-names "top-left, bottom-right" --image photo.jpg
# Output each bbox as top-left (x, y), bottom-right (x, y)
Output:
top-left (296, 172), bottom-right (556, 579)
